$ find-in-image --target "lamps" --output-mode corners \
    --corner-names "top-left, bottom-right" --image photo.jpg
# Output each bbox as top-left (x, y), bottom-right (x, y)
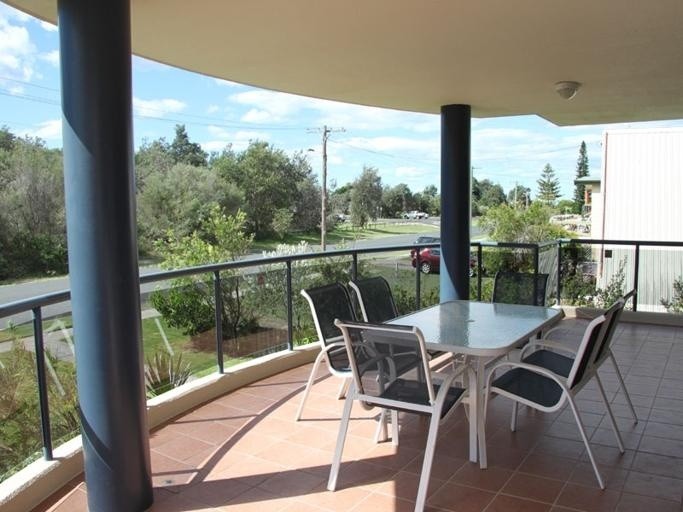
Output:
top-left (554, 82), bottom-right (581, 101)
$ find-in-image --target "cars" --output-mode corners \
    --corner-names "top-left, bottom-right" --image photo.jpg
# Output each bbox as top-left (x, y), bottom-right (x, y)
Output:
top-left (409, 210), bottom-right (429, 220)
top-left (410, 236), bottom-right (479, 279)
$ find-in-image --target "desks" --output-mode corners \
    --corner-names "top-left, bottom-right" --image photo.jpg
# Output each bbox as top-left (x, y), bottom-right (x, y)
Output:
top-left (369, 299), bottom-right (567, 470)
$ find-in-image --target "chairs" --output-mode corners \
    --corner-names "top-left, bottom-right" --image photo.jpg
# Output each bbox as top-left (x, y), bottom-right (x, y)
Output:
top-left (326, 317), bottom-right (480, 512)
top-left (490, 271), bottom-right (549, 368)
top-left (484, 314), bottom-right (625, 488)
top-left (348, 276), bottom-right (454, 424)
top-left (297, 280), bottom-right (394, 442)
top-left (540, 287), bottom-right (638, 455)
